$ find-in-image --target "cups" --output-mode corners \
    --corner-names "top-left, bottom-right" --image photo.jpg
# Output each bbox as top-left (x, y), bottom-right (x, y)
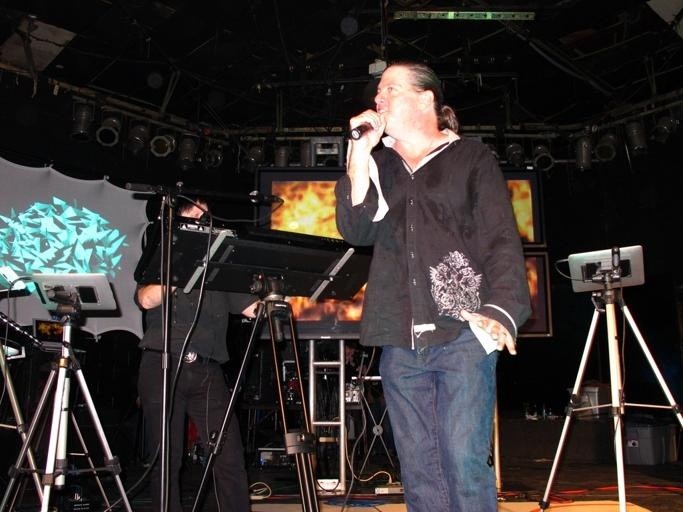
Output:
top-left (543, 406), bottom-right (551, 420)
top-left (526, 404), bottom-right (537, 419)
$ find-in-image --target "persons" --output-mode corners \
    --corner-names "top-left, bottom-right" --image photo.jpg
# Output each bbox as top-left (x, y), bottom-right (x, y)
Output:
top-left (332, 62), bottom-right (534, 511)
top-left (136, 194), bottom-right (266, 511)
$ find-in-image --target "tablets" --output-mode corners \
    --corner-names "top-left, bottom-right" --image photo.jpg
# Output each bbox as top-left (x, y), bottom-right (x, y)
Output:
top-left (568, 245), bottom-right (645, 293)
top-left (32, 273), bottom-right (117, 311)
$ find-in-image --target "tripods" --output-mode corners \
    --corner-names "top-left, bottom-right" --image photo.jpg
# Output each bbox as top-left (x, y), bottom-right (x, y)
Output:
top-left (539, 290), bottom-right (683, 512)
top-left (0, 305), bottom-right (134, 512)
top-left (191, 291), bottom-right (320, 512)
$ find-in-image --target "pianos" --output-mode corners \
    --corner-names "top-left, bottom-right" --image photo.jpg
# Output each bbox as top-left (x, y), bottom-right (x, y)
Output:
top-left (133, 215), bottom-right (373, 300)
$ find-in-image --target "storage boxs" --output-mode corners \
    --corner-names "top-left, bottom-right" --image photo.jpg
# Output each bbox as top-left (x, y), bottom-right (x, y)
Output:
top-left (624, 421), bottom-right (679, 466)
top-left (567, 387), bottom-right (611, 422)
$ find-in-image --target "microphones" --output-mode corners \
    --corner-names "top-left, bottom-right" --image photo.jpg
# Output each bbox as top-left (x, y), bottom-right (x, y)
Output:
top-left (350, 122), bottom-right (373, 140)
top-left (250, 190), bottom-right (281, 203)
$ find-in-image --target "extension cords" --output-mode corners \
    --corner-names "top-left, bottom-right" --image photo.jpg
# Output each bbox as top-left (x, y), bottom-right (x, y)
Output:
top-left (375, 483), bottom-right (405, 495)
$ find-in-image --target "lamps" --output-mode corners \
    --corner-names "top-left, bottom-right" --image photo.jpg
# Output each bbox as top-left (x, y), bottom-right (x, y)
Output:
top-left (73, 99), bottom-right (224, 173)
top-left (240, 119), bottom-right (594, 173)
top-left (598, 106), bottom-right (678, 162)
